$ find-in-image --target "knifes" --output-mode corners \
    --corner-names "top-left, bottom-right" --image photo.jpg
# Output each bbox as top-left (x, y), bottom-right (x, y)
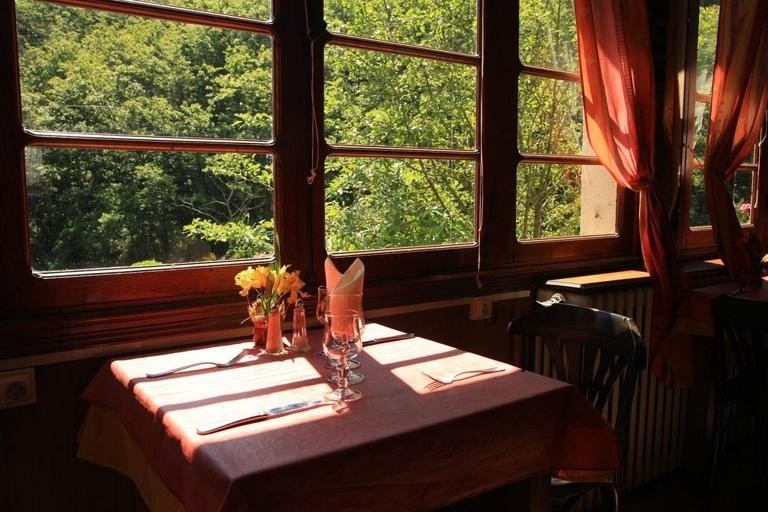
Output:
top-left (195, 396), bottom-right (332, 435)
top-left (357, 333), bottom-right (417, 348)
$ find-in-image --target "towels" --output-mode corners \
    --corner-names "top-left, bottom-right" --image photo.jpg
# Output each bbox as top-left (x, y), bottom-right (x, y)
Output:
top-left (323, 253), bottom-right (365, 333)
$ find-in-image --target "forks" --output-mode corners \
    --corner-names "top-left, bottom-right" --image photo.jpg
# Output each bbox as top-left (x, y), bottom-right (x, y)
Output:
top-left (420, 366), bottom-right (506, 386)
top-left (145, 348), bottom-right (248, 378)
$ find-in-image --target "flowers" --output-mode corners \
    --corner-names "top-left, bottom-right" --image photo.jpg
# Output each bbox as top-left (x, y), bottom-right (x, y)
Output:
top-left (737, 199), bottom-right (752, 226)
top-left (234, 256), bottom-right (309, 315)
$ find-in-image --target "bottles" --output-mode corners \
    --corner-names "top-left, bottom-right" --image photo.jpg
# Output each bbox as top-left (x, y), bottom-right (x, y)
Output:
top-left (290, 299), bottom-right (311, 353)
top-left (265, 304), bottom-right (284, 355)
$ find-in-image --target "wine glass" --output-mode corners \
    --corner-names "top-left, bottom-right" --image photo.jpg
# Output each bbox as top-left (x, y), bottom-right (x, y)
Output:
top-left (317, 285), bottom-right (330, 324)
top-left (324, 290), bottom-right (366, 401)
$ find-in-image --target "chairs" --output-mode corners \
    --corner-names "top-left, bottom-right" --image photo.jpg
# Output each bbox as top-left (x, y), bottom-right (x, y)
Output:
top-left (705, 290), bottom-right (767, 512)
top-left (501, 304), bottom-right (645, 510)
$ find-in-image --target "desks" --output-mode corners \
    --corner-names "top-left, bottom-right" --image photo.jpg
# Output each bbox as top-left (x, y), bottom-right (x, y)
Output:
top-left (80, 325), bottom-right (549, 511)
top-left (691, 278), bottom-right (767, 512)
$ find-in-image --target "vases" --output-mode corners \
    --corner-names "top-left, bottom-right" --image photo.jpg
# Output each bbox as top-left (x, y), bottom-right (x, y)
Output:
top-left (248, 309), bottom-right (269, 348)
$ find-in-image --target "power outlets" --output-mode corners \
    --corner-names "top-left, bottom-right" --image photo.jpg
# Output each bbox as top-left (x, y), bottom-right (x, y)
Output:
top-left (0, 367), bottom-right (37, 411)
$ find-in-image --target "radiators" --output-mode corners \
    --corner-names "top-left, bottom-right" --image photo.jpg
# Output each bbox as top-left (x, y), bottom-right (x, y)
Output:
top-left (536, 252), bottom-right (768, 512)
